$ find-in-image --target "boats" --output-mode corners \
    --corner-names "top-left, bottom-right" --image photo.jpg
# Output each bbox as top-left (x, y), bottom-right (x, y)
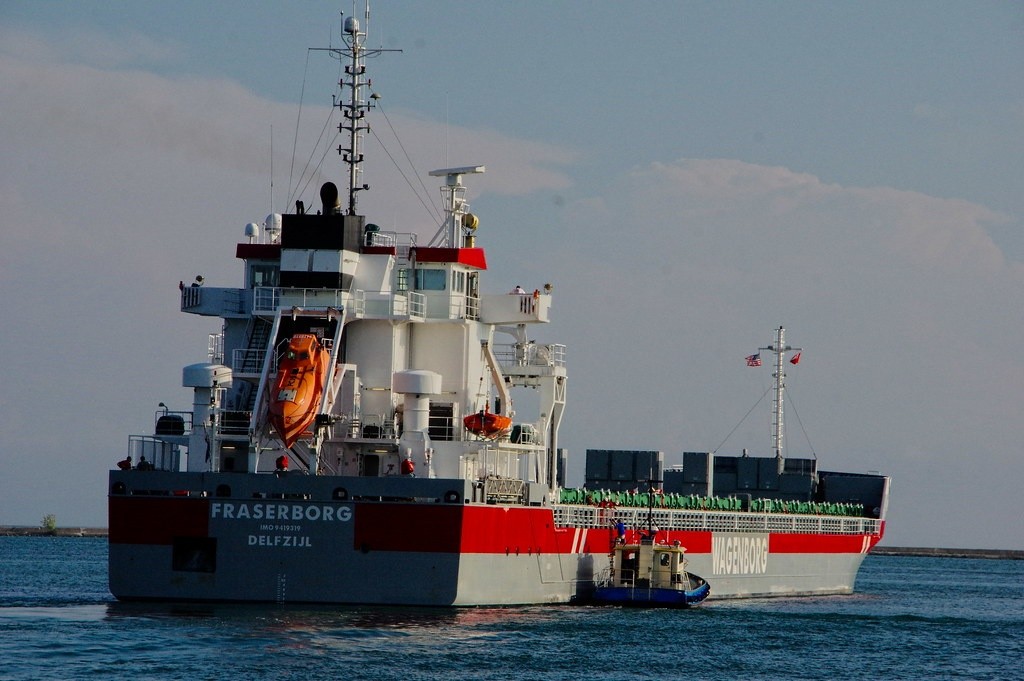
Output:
top-left (570, 447), bottom-right (711, 609)
top-left (108, 1), bottom-right (891, 611)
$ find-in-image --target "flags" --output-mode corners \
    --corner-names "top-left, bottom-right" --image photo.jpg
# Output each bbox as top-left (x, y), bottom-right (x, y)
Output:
top-left (745, 354), bottom-right (761, 366)
top-left (790, 352), bottom-right (800, 364)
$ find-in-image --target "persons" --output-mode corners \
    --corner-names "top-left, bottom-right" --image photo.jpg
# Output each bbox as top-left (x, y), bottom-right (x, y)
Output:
top-left (511, 286), bottom-right (525, 293)
top-left (117, 456), bottom-right (131, 470)
top-left (137, 456), bottom-right (149, 471)
